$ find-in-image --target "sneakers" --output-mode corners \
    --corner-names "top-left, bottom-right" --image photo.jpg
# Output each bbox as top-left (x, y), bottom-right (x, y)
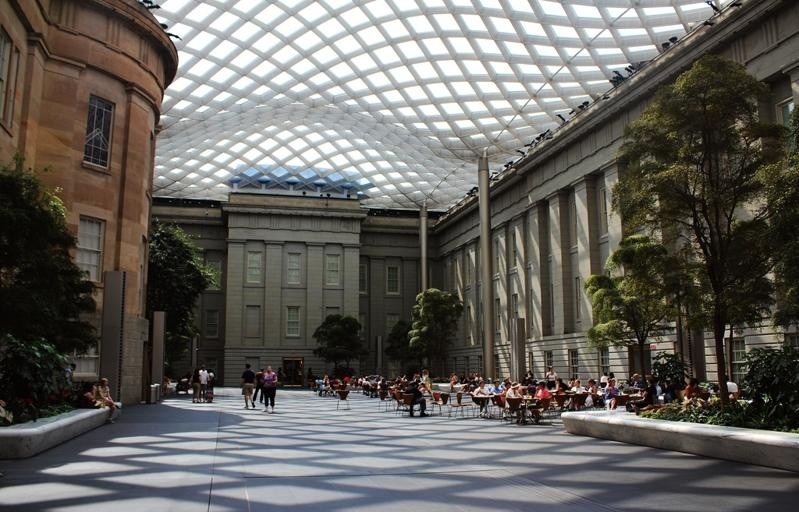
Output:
top-left (262, 408), bottom-right (275, 413)
top-left (244, 403), bottom-right (255, 409)
top-left (191, 400), bottom-right (213, 403)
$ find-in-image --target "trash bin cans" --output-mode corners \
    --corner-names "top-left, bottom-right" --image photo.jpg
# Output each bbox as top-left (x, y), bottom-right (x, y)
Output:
top-left (148, 383), bottom-right (160, 405)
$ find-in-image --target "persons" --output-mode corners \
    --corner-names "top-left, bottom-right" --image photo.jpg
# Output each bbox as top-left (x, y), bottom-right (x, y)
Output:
top-left (450, 366), bottom-right (738, 424)
top-left (79, 378), bottom-right (121, 424)
top-left (65, 363), bottom-right (76, 385)
top-left (192, 365), bottom-right (214, 403)
top-left (240, 363), bottom-right (277, 413)
top-left (315, 369), bottom-right (431, 417)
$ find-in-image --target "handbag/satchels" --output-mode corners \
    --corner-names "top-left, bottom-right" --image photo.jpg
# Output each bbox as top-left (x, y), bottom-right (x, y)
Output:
top-left (264, 383), bottom-right (270, 388)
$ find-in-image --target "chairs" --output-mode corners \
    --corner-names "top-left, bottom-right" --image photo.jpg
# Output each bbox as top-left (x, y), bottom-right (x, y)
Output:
top-left (315, 369), bottom-right (597, 425)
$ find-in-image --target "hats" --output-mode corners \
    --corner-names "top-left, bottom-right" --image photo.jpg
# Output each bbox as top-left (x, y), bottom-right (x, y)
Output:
top-left (493, 379), bottom-right (502, 385)
top-left (511, 382), bottom-right (519, 388)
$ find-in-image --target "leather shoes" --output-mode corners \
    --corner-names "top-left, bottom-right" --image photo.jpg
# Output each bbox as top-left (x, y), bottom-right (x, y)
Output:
top-left (409, 413), bottom-right (429, 417)
